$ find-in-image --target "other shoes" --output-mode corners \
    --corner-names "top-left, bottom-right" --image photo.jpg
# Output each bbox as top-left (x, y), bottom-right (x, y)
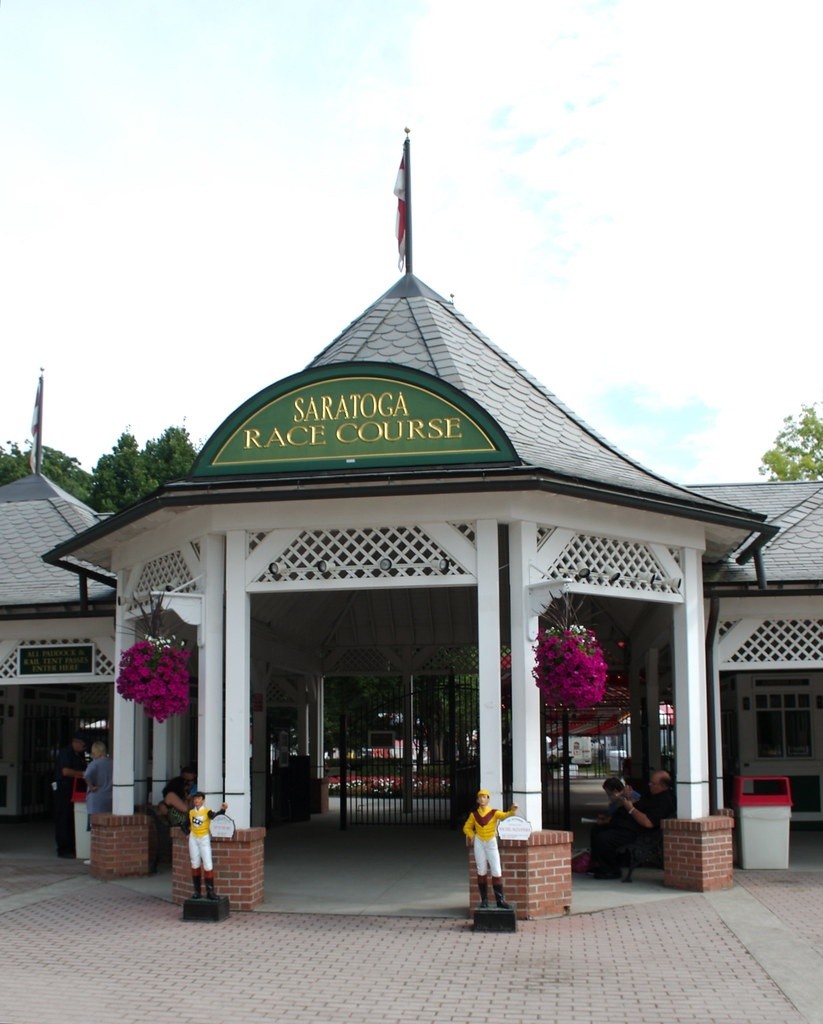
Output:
top-left (58, 852), bottom-right (76, 858)
top-left (594, 870), bottom-right (622, 879)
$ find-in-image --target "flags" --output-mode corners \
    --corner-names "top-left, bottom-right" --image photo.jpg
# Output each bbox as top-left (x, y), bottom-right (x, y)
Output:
top-left (393, 137), bottom-right (412, 274)
top-left (30, 377), bottom-right (42, 474)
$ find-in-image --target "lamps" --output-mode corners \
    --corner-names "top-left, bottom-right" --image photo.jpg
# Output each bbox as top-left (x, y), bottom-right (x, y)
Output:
top-left (638, 572), bottom-right (655, 584)
top-left (270, 561), bottom-right (287, 574)
top-left (604, 564), bottom-right (620, 581)
top-left (318, 559), bottom-right (336, 572)
top-left (431, 559), bottom-right (449, 572)
top-left (118, 594), bottom-right (134, 606)
top-left (165, 577), bottom-right (179, 592)
top-left (665, 576), bottom-right (681, 588)
top-left (578, 562), bottom-right (591, 578)
top-left (377, 558), bottom-right (391, 570)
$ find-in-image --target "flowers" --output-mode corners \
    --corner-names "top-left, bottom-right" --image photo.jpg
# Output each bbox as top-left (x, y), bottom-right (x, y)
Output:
top-left (531, 590), bottom-right (609, 709)
top-left (117, 591), bottom-right (192, 723)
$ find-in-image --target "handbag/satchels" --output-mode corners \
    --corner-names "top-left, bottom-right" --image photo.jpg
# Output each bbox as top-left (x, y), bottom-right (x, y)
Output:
top-left (71, 773), bottom-right (87, 802)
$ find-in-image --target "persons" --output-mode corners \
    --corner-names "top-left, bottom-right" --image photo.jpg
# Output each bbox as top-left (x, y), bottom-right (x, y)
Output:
top-left (46, 732), bottom-right (113, 859)
top-left (463, 790), bottom-right (519, 909)
top-left (181, 792), bottom-right (229, 900)
top-left (157, 765), bottom-right (197, 845)
top-left (585, 769), bottom-right (678, 883)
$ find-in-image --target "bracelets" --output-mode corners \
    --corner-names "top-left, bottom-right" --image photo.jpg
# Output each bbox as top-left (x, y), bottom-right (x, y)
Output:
top-left (629, 807), bottom-right (636, 814)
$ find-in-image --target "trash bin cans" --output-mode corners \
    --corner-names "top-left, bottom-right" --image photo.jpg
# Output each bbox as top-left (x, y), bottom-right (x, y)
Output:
top-left (732, 776), bottom-right (793, 871)
top-left (70, 773), bottom-right (91, 859)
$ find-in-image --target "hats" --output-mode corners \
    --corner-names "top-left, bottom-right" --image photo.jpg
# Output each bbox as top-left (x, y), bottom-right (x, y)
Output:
top-left (72, 731), bottom-right (88, 740)
